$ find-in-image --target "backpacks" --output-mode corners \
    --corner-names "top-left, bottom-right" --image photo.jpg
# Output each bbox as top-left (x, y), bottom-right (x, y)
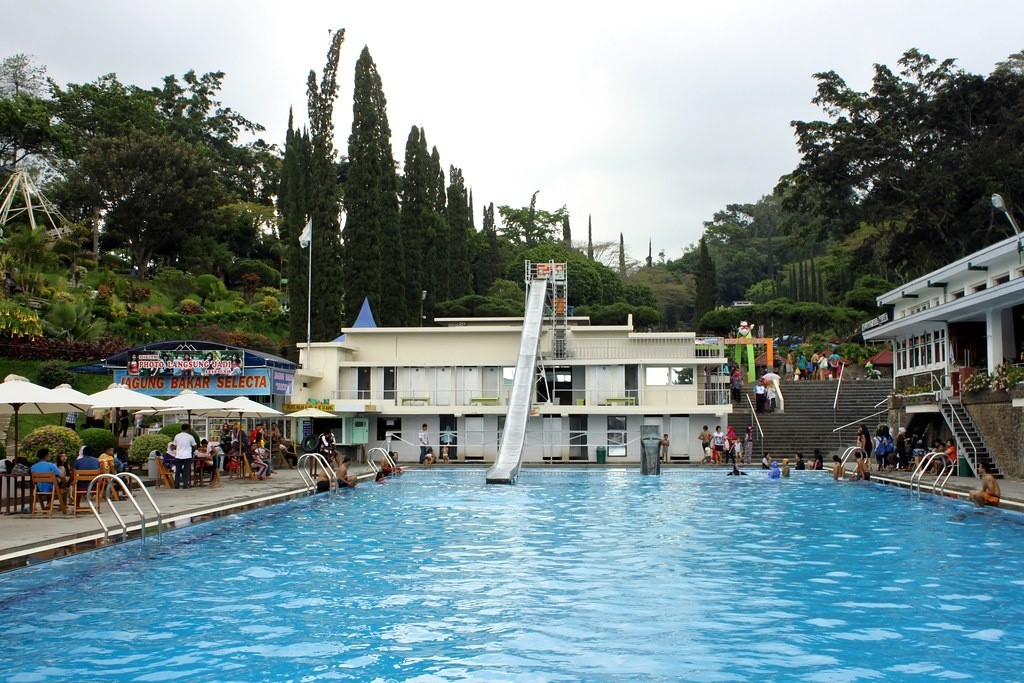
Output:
top-left (884, 435), bottom-right (894, 453)
top-left (875, 436), bottom-right (886, 456)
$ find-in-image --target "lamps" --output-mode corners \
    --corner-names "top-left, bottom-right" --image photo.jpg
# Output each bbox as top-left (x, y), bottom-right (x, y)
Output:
top-left (991, 193), bottom-right (1024, 255)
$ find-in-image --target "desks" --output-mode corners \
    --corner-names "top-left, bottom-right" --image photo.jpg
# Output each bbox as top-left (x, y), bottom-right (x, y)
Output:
top-left (219, 452), bottom-right (246, 480)
top-left (110, 463), bottom-right (133, 494)
top-left (60, 469), bottom-right (73, 505)
top-left (167, 456), bottom-right (205, 485)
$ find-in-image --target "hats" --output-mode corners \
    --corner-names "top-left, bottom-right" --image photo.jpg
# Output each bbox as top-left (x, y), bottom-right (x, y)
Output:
top-left (773, 461), bottom-right (778, 468)
top-left (783, 458), bottom-right (789, 463)
top-left (221, 354), bottom-right (229, 361)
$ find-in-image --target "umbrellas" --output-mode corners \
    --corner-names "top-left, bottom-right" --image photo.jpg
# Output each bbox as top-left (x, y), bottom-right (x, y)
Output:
top-left (773, 335), bottom-right (802, 345)
top-left (0, 373), bottom-right (338, 467)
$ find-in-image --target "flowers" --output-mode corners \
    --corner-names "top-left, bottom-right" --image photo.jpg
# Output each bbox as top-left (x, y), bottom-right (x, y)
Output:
top-left (889, 359), bottom-right (1024, 409)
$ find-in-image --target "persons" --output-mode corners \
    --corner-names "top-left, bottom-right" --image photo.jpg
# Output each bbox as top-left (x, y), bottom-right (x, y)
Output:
top-left (128, 353), bottom-right (244, 376)
top-left (661, 350), bottom-right (1024, 508)
top-left (0, 407), bottom-right (362, 510)
top-left (375, 423), bottom-right (449, 484)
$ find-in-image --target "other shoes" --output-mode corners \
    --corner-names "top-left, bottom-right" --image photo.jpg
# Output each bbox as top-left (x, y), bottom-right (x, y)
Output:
top-left (258, 477), bottom-right (263, 480)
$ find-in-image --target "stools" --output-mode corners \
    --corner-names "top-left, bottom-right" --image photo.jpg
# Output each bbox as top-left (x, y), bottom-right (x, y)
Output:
top-left (576, 398), bottom-right (584, 406)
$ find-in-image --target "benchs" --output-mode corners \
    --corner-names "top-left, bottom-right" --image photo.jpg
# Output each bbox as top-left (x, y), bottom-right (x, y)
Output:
top-left (470, 397), bottom-right (499, 405)
top-left (605, 397), bottom-right (635, 406)
top-left (909, 453), bottom-right (957, 476)
top-left (400, 397), bottom-right (430, 405)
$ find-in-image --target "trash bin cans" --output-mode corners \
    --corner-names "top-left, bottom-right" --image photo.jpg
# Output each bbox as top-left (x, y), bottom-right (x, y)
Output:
top-left (959, 455), bottom-right (970, 477)
top-left (148, 450), bottom-right (163, 479)
top-left (596, 446), bottom-right (606, 464)
top-left (214, 446), bottom-right (225, 471)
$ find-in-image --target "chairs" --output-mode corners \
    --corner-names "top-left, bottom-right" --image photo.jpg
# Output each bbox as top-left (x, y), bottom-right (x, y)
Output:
top-left (31, 438), bottom-right (302, 517)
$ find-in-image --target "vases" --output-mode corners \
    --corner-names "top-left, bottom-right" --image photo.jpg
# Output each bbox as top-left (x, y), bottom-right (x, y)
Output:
top-left (892, 392), bottom-right (935, 408)
top-left (960, 383), bottom-right (1024, 405)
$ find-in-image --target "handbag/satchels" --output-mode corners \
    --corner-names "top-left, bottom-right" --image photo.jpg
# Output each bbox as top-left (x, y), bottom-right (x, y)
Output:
top-left (831, 359), bottom-right (839, 367)
